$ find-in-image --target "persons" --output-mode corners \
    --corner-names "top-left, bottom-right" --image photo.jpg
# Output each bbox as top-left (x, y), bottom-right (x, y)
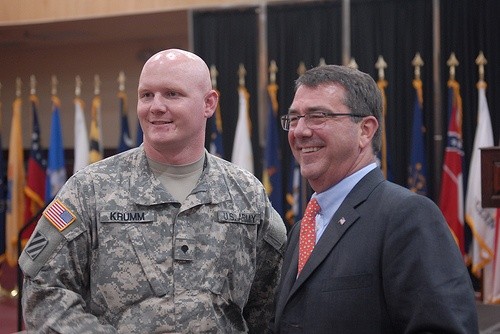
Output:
top-left (18, 49), bottom-right (288, 334)
top-left (274, 65), bottom-right (481, 334)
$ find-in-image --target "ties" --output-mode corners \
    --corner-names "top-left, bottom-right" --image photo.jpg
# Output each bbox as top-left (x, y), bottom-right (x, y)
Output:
top-left (297, 197), bottom-right (321, 279)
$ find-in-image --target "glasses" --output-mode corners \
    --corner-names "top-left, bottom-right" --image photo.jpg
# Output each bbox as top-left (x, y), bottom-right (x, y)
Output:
top-left (279, 111), bottom-right (365, 131)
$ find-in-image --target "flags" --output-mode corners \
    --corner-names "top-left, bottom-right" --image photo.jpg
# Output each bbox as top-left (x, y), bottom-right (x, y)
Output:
top-left (5, 78), bottom-right (500, 304)
